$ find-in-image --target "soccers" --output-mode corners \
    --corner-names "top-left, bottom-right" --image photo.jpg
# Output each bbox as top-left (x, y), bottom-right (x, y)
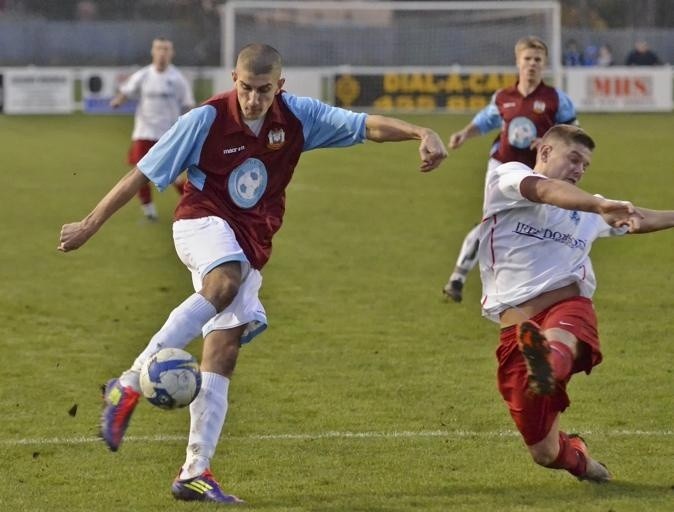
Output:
top-left (235, 165), bottom-right (263, 199)
top-left (512, 123), bottom-right (533, 143)
top-left (138, 347), bottom-right (201, 410)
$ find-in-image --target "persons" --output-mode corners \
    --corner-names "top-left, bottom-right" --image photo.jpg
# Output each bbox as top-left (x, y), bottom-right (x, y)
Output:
top-left (561, 36), bottom-right (665, 69)
top-left (108, 36), bottom-right (197, 228)
top-left (443, 34), bottom-right (578, 302)
top-left (53, 43), bottom-right (451, 502)
top-left (477, 127), bottom-right (673, 480)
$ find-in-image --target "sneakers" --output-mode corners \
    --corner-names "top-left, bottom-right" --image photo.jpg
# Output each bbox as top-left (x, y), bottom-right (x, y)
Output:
top-left (570, 435), bottom-right (607, 482)
top-left (516, 319), bottom-right (554, 397)
top-left (441, 279), bottom-right (463, 301)
top-left (98, 379), bottom-right (138, 449)
top-left (172, 466), bottom-right (236, 501)
